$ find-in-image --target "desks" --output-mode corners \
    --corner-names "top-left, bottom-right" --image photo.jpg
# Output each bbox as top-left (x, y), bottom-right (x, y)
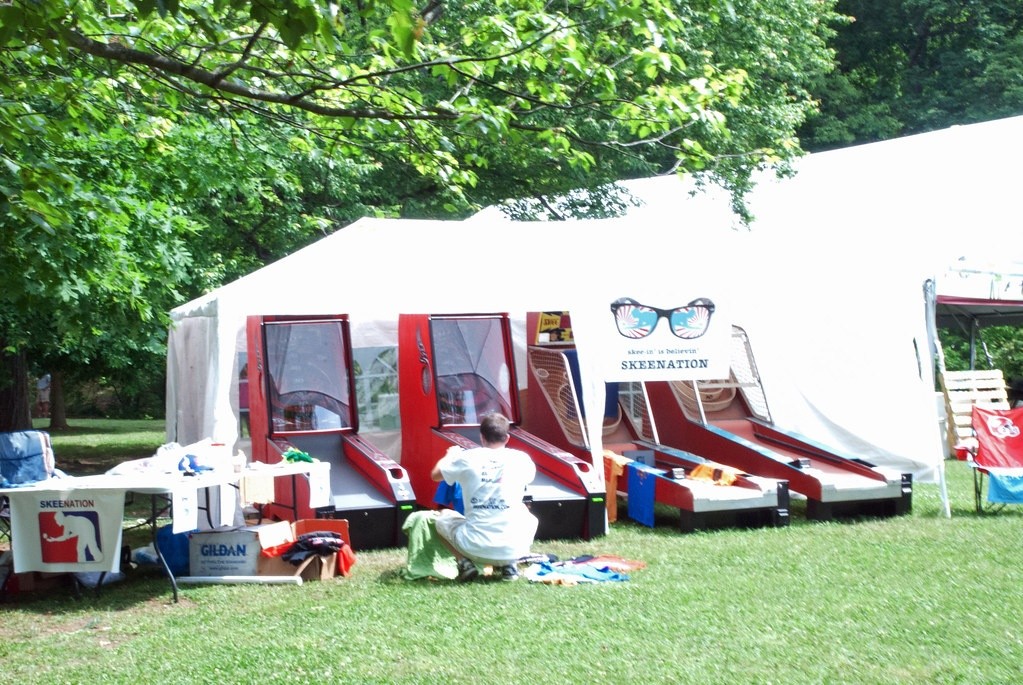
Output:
top-left (0, 458), bottom-right (332, 607)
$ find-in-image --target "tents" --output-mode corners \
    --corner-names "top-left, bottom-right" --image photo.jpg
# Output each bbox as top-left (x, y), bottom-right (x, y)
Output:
top-left (162, 107), bottom-right (1023, 519)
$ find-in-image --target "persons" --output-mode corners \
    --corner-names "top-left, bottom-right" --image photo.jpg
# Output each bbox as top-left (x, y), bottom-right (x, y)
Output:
top-left (34, 372), bottom-right (52, 419)
top-left (432, 412), bottom-right (540, 583)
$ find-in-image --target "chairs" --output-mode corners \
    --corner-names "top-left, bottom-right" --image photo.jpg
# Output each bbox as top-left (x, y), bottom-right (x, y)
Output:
top-left (0, 430), bottom-right (73, 547)
top-left (970, 404), bottom-right (1023, 516)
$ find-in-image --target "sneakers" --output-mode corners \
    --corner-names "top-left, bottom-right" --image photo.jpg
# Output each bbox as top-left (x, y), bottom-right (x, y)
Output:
top-left (506, 564), bottom-right (521, 581)
top-left (456, 557), bottom-right (476, 580)
